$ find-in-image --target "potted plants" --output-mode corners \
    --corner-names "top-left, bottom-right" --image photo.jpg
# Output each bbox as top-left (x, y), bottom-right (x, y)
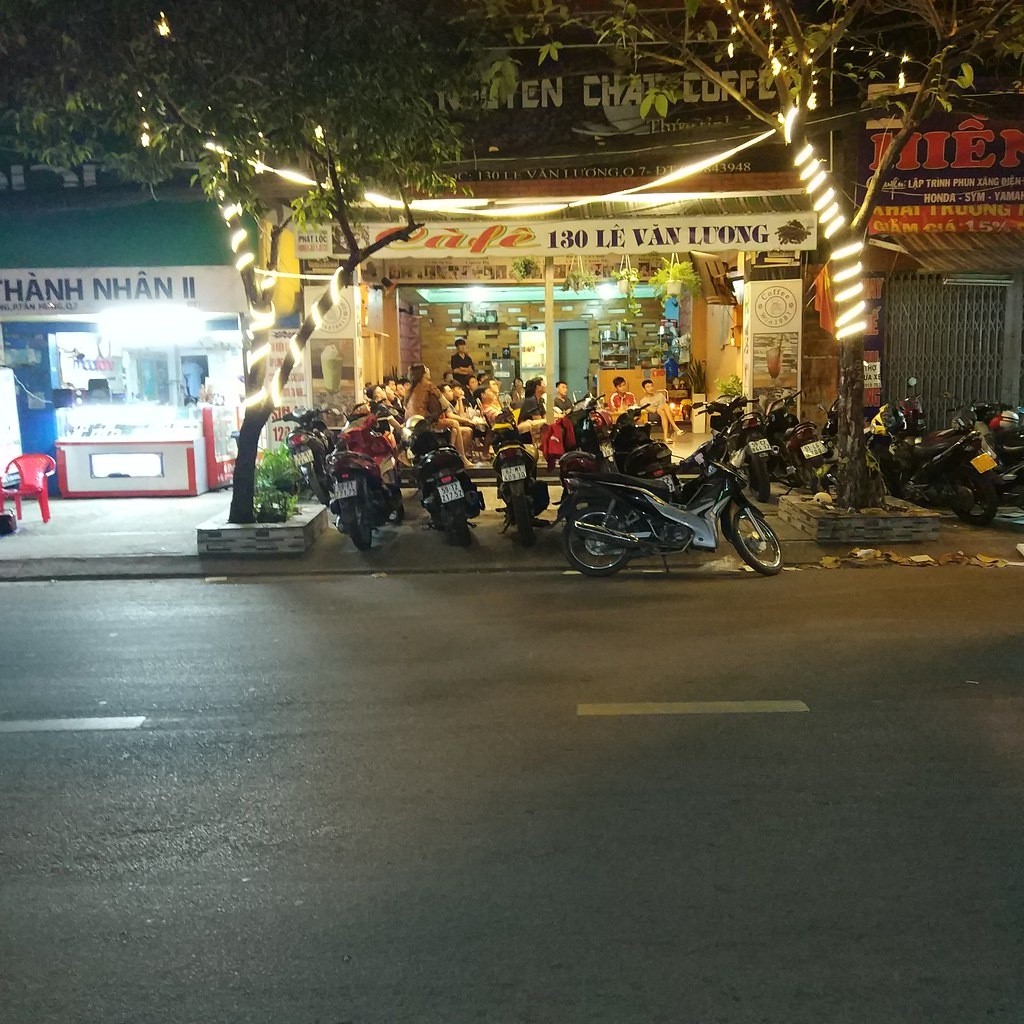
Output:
top-left (649, 257), bottom-right (701, 307)
top-left (648, 346), bottom-right (662, 365)
top-left (610, 268), bottom-right (640, 315)
top-left (678, 359), bottom-right (707, 434)
top-left (714, 373), bottom-right (742, 412)
top-left (560, 270), bottom-right (598, 294)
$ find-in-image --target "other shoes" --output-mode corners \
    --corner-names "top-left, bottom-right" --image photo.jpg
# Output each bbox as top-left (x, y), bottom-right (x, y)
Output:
top-left (397, 454), bottom-right (411, 467)
top-left (664, 438), bottom-right (673, 444)
top-left (674, 430), bottom-right (686, 437)
top-left (465, 447), bottom-right (492, 463)
top-left (461, 457), bottom-right (476, 467)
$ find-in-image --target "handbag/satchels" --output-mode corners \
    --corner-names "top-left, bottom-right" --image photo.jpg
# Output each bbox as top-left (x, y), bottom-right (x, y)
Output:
top-left (0, 509), bottom-right (17, 534)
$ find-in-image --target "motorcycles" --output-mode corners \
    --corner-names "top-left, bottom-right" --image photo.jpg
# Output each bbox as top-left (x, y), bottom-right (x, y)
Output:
top-left (478, 393), bottom-right (551, 547)
top-left (606, 402), bottom-right (676, 479)
top-left (403, 408), bottom-right (485, 547)
top-left (763, 391), bottom-right (847, 496)
top-left (273, 406), bottom-right (345, 506)
top-left (540, 393), bottom-right (611, 491)
top-left (326, 398), bottom-right (405, 552)
top-left (817, 359), bottom-right (1024, 526)
top-left (692, 394), bottom-right (780, 503)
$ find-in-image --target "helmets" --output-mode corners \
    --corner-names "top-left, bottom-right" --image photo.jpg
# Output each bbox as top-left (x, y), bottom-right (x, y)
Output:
top-left (588, 410), bottom-right (613, 434)
top-left (990, 410), bottom-right (1019, 433)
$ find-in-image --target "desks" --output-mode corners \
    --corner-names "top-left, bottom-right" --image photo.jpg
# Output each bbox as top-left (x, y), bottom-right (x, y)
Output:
top-left (609, 405), bottom-right (648, 425)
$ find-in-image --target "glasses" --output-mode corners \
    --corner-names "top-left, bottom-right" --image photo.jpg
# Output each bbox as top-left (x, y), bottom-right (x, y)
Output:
top-left (378, 388), bottom-right (384, 394)
top-left (425, 368), bottom-right (429, 372)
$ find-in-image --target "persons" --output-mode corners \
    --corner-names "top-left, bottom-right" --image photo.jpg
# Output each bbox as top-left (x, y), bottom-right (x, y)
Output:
top-left (607, 376), bottom-right (637, 410)
top-left (364, 376), bottom-right (411, 467)
top-left (449, 339), bottom-right (475, 385)
top-left (510, 378), bottom-right (526, 403)
top-left (403, 364), bottom-right (476, 468)
top-left (553, 382), bottom-right (573, 412)
top-left (472, 379), bottom-right (533, 437)
top-left (433, 371), bottom-right (493, 468)
top-left (638, 380), bottom-right (684, 444)
top-left (179, 356), bottom-right (205, 406)
top-left (516, 376), bottom-right (547, 461)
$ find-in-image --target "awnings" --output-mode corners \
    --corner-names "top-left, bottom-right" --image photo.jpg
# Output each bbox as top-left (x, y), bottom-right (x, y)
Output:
top-left (0, 202), bottom-right (259, 315)
top-left (279, 184), bottom-right (815, 260)
top-left (883, 230), bottom-right (1024, 279)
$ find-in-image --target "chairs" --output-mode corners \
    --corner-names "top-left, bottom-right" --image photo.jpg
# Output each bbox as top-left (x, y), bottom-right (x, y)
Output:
top-left (0, 454), bottom-right (56, 523)
top-left (475, 389), bottom-right (671, 459)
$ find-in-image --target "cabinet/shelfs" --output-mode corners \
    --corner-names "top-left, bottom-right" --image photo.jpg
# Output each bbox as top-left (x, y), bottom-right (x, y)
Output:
top-left (599, 332), bottom-right (631, 371)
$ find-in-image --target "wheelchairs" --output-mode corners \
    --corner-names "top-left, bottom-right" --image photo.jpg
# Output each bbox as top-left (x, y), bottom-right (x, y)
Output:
top-left (546, 426), bottom-right (785, 577)
